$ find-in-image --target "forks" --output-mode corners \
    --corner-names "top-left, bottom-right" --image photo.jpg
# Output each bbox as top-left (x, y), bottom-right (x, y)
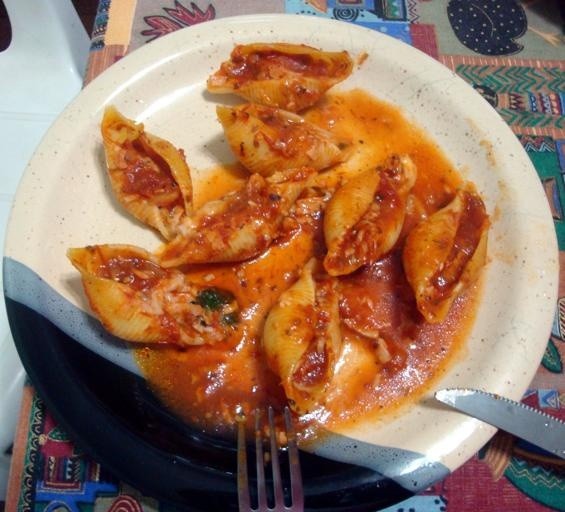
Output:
top-left (3, 14), bottom-right (560, 506)
top-left (235, 407), bottom-right (303, 511)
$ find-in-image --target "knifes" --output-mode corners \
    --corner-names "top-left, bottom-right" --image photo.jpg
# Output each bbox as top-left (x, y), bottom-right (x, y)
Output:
top-left (432, 387), bottom-right (565, 461)
